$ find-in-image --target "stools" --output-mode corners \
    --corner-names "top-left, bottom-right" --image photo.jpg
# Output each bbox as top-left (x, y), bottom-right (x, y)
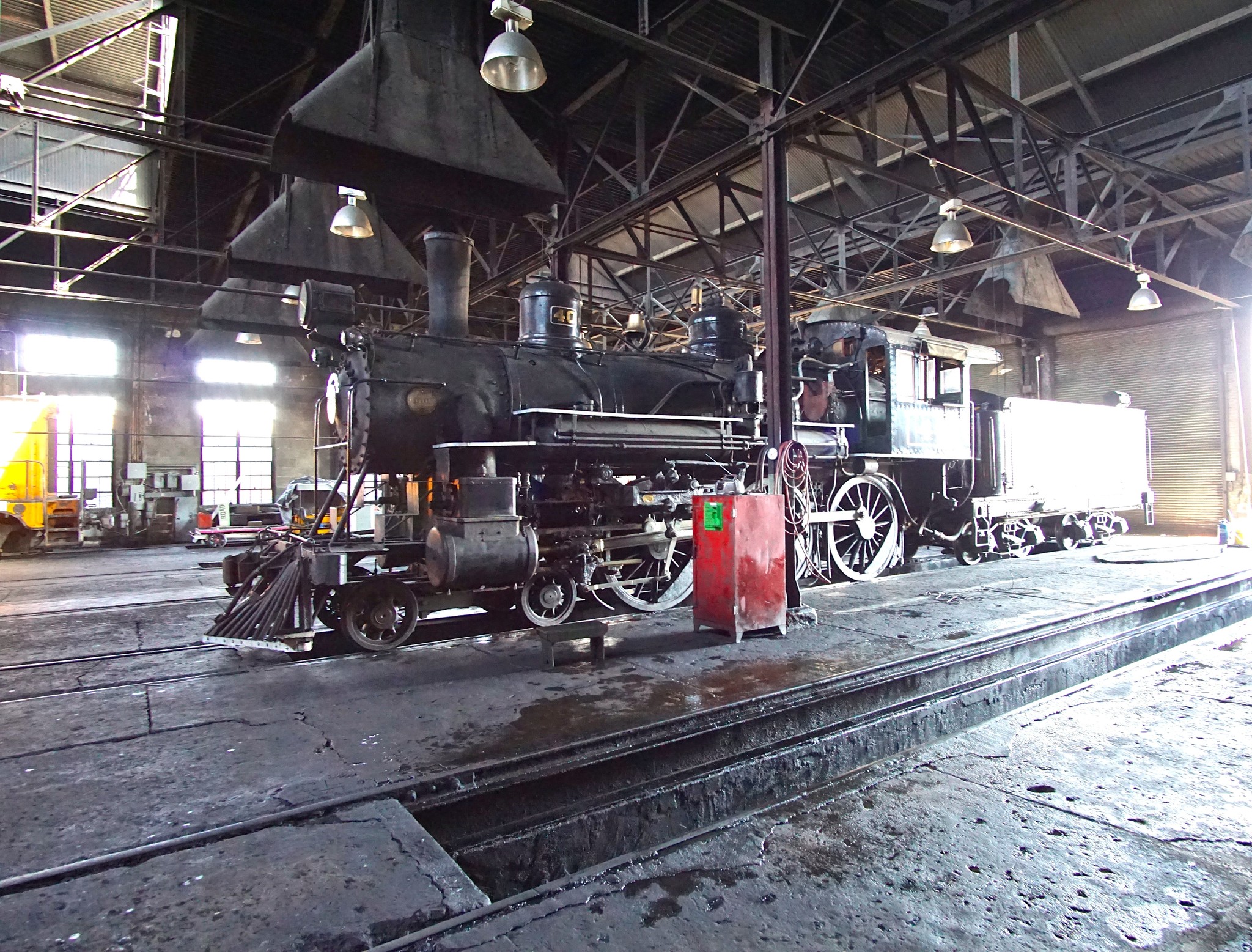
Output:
top-left (536, 620), bottom-right (610, 668)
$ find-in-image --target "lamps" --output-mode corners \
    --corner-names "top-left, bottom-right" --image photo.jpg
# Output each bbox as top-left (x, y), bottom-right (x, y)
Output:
top-left (480, 0), bottom-right (548, 94)
top-left (281, 284), bottom-right (301, 305)
top-left (329, 185), bottom-right (375, 238)
top-left (1127, 274), bottom-right (1163, 312)
top-left (929, 198), bottom-right (974, 254)
top-left (996, 360), bottom-right (1015, 375)
top-left (913, 319), bottom-right (932, 336)
top-left (621, 307), bottom-right (653, 339)
top-left (235, 332), bottom-right (262, 345)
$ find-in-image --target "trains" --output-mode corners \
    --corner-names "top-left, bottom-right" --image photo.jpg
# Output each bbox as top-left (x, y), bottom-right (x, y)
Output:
top-left (214, 228), bottom-right (1156, 653)
